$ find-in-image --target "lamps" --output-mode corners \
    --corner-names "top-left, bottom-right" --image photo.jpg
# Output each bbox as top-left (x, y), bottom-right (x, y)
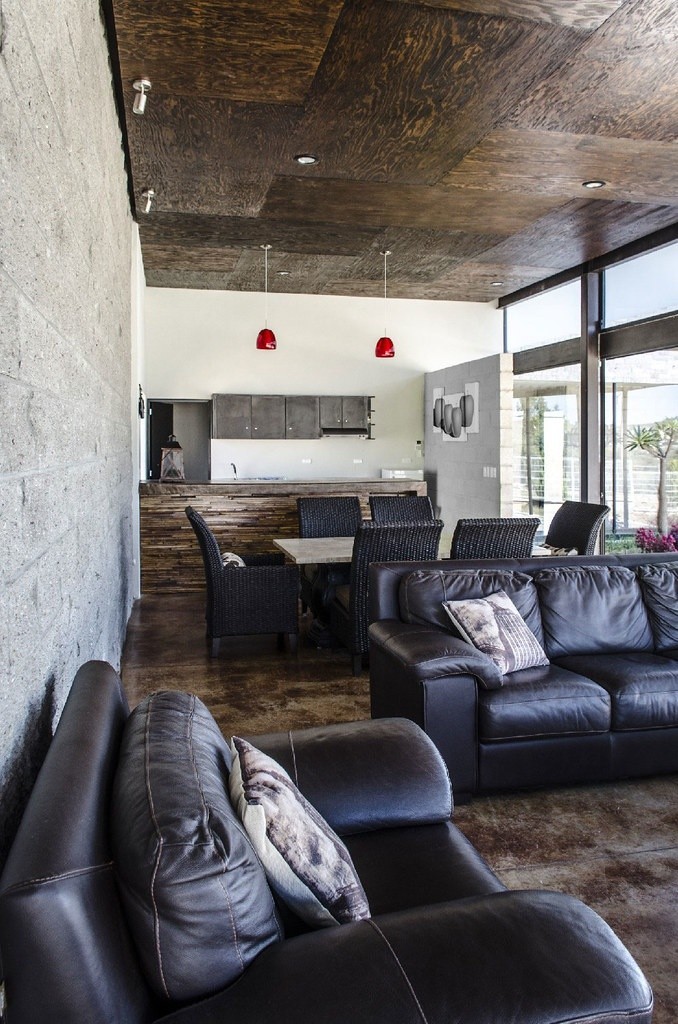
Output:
top-left (133, 79), bottom-right (152, 115)
top-left (375, 250), bottom-right (395, 358)
top-left (141, 188), bottom-right (155, 215)
top-left (256, 244), bottom-right (277, 351)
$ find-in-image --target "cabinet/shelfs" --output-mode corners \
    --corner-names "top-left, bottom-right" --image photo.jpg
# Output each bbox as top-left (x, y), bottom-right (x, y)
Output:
top-left (272, 533), bottom-right (552, 648)
top-left (209, 393), bottom-right (377, 440)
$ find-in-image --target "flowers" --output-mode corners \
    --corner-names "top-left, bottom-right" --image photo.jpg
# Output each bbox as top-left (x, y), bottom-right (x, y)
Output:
top-left (635, 524), bottom-right (678, 554)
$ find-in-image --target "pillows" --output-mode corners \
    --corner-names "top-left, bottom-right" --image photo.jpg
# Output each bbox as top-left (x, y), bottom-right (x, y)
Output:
top-left (219, 552), bottom-right (248, 568)
top-left (441, 588), bottom-right (551, 676)
top-left (229, 734), bottom-right (372, 929)
top-left (539, 543), bottom-right (578, 555)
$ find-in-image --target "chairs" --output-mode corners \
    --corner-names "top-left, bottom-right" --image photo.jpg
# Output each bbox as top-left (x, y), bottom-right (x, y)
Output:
top-left (545, 500), bottom-right (612, 555)
top-left (369, 496), bottom-right (435, 520)
top-left (185, 506), bottom-right (300, 658)
top-left (296, 496), bottom-right (362, 618)
top-left (329, 519), bottom-right (444, 677)
top-left (442, 517), bottom-right (540, 559)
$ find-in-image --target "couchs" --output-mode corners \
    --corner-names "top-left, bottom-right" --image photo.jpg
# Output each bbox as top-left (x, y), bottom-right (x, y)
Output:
top-left (366, 552), bottom-right (678, 806)
top-left (0, 661), bottom-right (656, 1024)
